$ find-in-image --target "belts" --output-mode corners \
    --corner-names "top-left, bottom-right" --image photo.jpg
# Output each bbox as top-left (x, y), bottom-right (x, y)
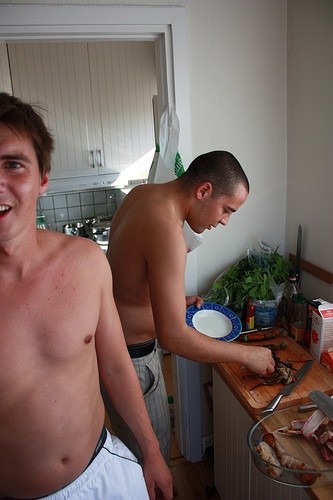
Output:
top-left (128, 341), bottom-right (156, 358)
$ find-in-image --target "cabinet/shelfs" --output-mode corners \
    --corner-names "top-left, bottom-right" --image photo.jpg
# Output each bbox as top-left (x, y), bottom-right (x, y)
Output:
top-left (0, 41), bottom-right (163, 190)
top-left (211, 366), bottom-right (311, 500)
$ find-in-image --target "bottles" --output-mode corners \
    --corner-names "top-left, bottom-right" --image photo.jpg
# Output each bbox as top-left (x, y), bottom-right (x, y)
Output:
top-left (280, 274), bottom-right (321, 351)
top-left (243, 297), bottom-right (255, 331)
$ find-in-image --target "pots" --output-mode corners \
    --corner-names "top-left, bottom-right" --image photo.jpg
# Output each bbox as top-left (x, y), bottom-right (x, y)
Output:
top-left (63, 214), bottom-right (115, 243)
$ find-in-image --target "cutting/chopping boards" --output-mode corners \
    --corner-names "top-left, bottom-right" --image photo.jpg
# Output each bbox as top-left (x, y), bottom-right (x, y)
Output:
top-left (217, 331), bottom-right (333, 415)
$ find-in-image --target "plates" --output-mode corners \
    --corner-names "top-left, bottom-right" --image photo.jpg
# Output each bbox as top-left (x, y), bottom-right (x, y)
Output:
top-left (185, 303), bottom-right (242, 342)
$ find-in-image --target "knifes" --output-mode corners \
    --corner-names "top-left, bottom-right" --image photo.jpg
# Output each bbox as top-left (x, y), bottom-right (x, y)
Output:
top-left (263, 360), bottom-right (312, 412)
top-left (308, 389), bottom-right (333, 422)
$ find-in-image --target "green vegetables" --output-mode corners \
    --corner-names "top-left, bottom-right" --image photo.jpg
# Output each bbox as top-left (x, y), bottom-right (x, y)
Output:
top-left (201, 242), bottom-right (294, 310)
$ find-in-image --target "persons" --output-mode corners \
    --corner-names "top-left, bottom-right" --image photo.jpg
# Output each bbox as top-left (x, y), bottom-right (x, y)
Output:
top-left (100, 150), bottom-right (276, 500)
top-left (0, 90), bottom-right (173, 500)
